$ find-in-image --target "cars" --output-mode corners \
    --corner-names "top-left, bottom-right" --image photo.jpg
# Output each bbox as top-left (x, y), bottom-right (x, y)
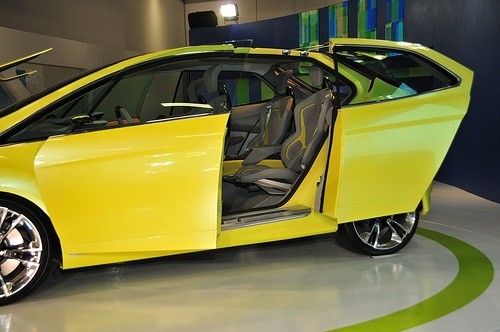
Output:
top-left (0, 37), bottom-right (475, 306)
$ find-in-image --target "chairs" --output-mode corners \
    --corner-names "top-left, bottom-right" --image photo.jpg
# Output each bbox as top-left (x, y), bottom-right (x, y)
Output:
top-left (186, 65), bottom-right (332, 194)
